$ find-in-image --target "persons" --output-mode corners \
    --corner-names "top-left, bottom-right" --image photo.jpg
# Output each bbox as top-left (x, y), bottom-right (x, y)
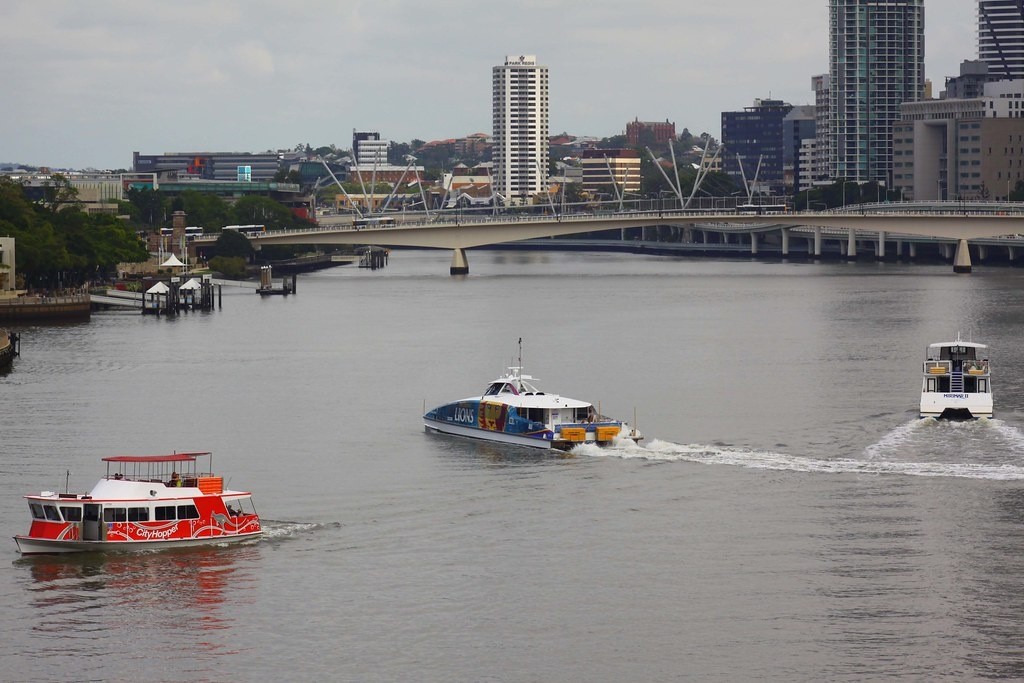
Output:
top-left (115, 472), bottom-right (126, 481)
top-left (237, 508), bottom-right (243, 516)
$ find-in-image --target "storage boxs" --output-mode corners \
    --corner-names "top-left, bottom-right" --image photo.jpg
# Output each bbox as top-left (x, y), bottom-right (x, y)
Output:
top-left (595, 427), bottom-right (621, 441)
top-left (968, 370), bottom-right (984, 374)
top-left (560, 428), bottom-right (585, 440)
top-left (930, 367), bottom-right (945, 374)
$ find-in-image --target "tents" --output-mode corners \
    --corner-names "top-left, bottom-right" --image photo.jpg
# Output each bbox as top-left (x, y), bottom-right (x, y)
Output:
top-left (161, 253), bottom-right (189, 274)
top-left (179, 278), bottom-right (201, 304)
top-left (145, 282), bottom-right (169, 307)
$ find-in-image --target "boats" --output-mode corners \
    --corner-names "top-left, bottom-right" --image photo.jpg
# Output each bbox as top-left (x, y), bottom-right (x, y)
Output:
top-left (919, 329), bottom-right (993, 419)
top-left (0, 327), bottom-right (21, 364)
top-left (422, 336), bottom-right (644, 451)
top-left (13, 450), bottom-right (265, 555)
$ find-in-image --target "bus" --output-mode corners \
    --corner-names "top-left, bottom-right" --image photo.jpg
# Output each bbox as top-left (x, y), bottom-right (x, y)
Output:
top-left (159, 227), bottom-right (203, 239)
top-left (736, 204), bottom-right (787, 215)
top-left (222, 225), bottom-right (265, 237)
top-left (352, 217), bottom-right (395, 229)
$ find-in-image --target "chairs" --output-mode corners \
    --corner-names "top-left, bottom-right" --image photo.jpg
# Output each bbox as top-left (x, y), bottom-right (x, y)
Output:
top-left (139, 478), bottom-right (197, 487)
